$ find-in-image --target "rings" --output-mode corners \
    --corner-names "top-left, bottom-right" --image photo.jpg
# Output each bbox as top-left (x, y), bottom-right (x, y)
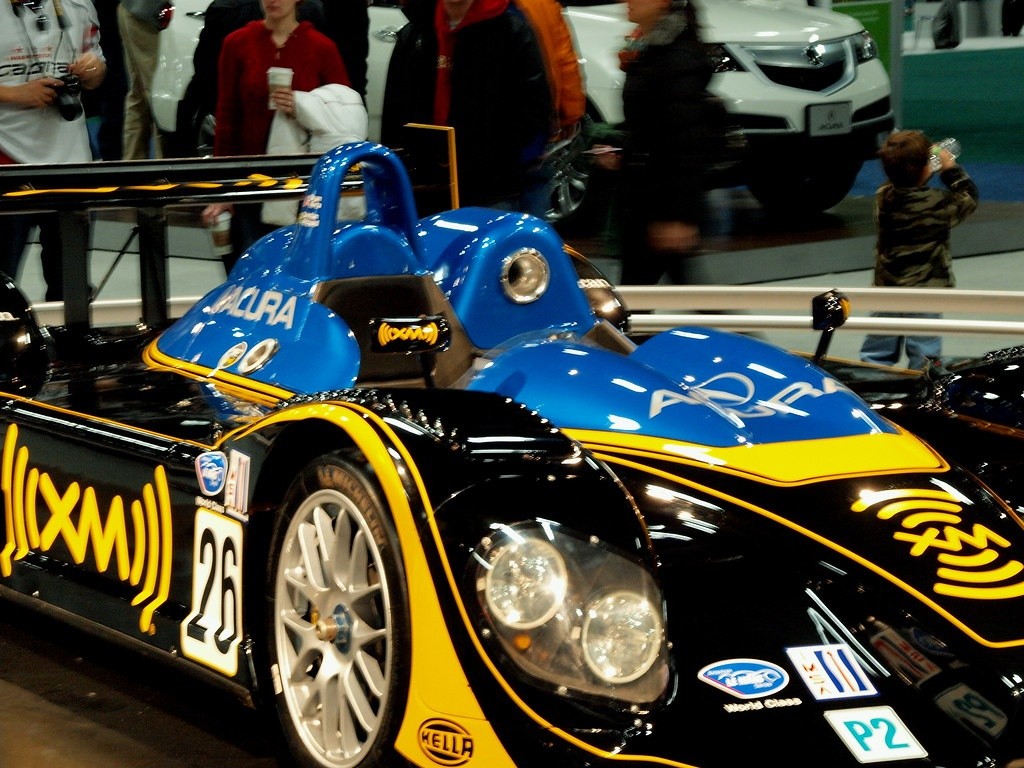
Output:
top-left (289, 101), bottom-right (291, 105)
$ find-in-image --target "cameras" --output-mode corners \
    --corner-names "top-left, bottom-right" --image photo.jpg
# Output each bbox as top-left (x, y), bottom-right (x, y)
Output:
top-left (45, 73), bottom-right (86, 122)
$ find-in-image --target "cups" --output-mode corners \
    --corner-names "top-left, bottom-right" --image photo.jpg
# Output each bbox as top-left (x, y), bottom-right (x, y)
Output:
top-left (265, 66), bottom-right (294, 110)
top-left (207, 211), bottom-right (233, 257)
top-left (926, 136), bottom-right (961, 173)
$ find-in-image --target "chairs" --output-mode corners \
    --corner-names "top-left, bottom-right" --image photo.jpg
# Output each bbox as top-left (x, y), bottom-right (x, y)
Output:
top-left (315, 274), bottom-right (473, 390)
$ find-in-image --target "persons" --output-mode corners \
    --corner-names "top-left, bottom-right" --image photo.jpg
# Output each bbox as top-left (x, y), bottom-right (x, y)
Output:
top-left (858, 128), bottom-right (980, 372)
top-left (591, 0), bottom-right (747, 335)
top-left (0, 0), bottom-right (587, 303)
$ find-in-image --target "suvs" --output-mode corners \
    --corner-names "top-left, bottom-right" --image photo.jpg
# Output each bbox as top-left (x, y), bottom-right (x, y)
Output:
top-left (147, 0), bottom-right (898, 247)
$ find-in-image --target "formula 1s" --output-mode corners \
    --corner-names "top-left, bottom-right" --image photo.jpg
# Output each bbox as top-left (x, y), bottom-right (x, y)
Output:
top-left (0, 143), bottom-right (1022, 768)
